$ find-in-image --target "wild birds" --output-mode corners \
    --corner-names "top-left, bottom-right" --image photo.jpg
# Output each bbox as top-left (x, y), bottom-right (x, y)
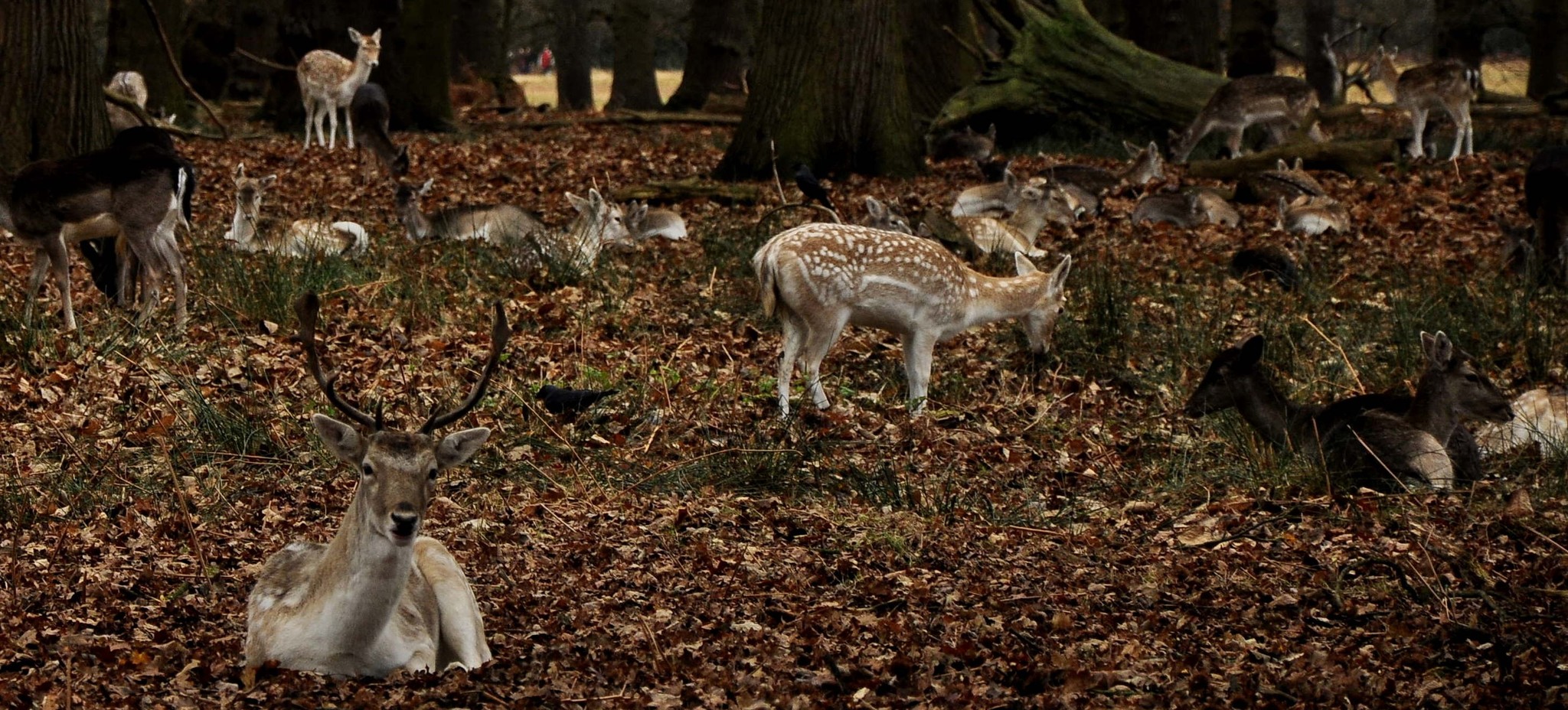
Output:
top-left (534, 383), bottom-right (622, 424)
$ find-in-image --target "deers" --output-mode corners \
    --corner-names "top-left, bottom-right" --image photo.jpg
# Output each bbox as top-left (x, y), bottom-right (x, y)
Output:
top-left (0, 25), bottom-right (688, 335)
top-left (246, 288), bottom-right (506, 682)
top-left (750, 18), bottom-right (1568, 496)
